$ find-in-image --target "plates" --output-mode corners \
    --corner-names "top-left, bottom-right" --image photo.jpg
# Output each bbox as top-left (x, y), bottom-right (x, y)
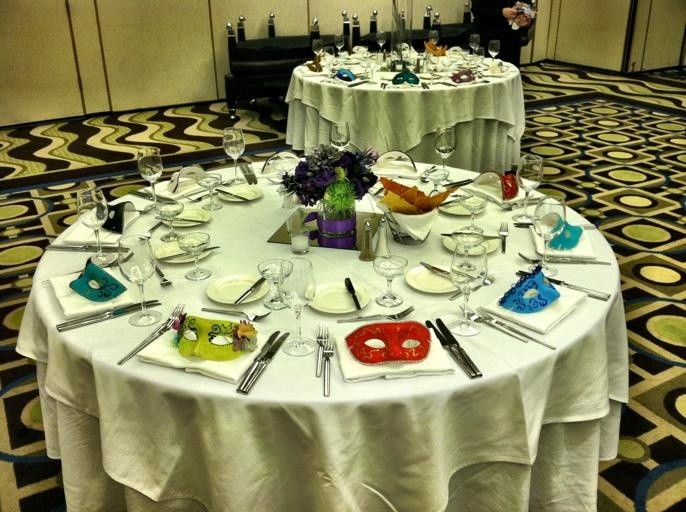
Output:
top-left (162, 210), bottom-right (210, 226)
top-left (438, 200), bottom-right (485, 214)
top-left (307, 283), bottom-right (370, 314)
top-left (219, 185), bottom-right (263, 202)
top-left (156, 240), bottom-right (211, 263)
top-left (443, 237), bottom-right (499, 257)
top-left (206, 274), bottom-right (270, 305)
top-left (406, 264), bottom-right (460, 293)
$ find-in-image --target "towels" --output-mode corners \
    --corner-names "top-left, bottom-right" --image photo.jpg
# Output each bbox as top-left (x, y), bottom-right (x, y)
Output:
top-left (528, 223), bottom-right (594, 261)
top-left (371, 163), bottom-right (436, 180)
top-left (333, 321), bottom-right (456, 382)
top-left (60, 206), bottom-right (140, 241)
top-left (142, 180), bottom-right (207, 203)
top-left (390, 206), bottom-right (440, 243)
top-left (486, 279), bottom-right (588, 336)
top-left (48, 263), bottom-right (146, 315)
top-left (134, 317), bottom-right (272, 384)
top-left (460, 180), bottom-right (547, 209)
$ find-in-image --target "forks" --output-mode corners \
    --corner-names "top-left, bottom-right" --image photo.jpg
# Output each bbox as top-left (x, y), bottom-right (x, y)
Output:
top-left (201, 306), bottom-right (273, 322)
top-left (315, 324), bottom-right (336, 398)
top-left (457, 302), bottom-right (558, 351)
top-left (118, 304), bottom-right (186, 365)
top-left (499, 221), bottom-right (509, 254)
top-left (517, 251), bottom-right (612, 266)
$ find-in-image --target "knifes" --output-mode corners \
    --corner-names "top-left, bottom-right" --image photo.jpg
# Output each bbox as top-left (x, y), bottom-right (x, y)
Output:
top-left (420, 262), bottom-right (452, 276)
top-left (443, 178), bottom-right (474, 189)
top-left (128, 189), bottom-right (178, 205)
top-left (156, 245), bottom-right (221, 261)
top-left (215, 188), bottom-right (250, 202)
top-left (440, 194), bottom-right (474, 206)
top-left (440, 233), bottom-right (499, 239)
top-left (239, 162), bottom-right (257, 186)
top-left (234, 276), bottom-right (265, 307)
top-left (345, 277), bottom-right (362, 311)
top-left (54, 299), bottom-right (161, 332)
top-left (426, 318), bottom-right (483, 378)
top-left (236, 331), bottom-right (289, 395)
top-left (516, 270), bottom-right (611, 300)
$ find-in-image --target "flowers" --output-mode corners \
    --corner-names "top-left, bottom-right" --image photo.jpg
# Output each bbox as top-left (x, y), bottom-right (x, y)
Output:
top-left (278, 139), bottom-right (380, 216)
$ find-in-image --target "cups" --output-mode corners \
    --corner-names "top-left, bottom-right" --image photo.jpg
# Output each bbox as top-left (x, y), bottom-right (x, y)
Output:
top-left (289, 224), bottom-right (311, 253)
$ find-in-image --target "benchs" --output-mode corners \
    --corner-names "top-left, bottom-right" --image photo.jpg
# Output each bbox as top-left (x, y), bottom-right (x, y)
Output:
top-left (225, 1), bottom-right (476, 120)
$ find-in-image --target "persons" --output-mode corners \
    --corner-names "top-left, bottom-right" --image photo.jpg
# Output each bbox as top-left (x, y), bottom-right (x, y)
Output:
top-left (471, 1), bottom-right (536, 71)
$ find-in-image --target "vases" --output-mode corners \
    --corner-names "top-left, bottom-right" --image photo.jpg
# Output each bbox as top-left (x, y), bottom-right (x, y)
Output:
top-left (309, 229), bottom-right (357, 250)
top-left (303, 177), bottom-right (358, 237)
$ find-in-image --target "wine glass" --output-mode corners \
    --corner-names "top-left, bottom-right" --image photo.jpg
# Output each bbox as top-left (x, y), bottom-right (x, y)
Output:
top-left (449, 240), bottom-right (486, 337)
top-left (373, 255), bottom-right (407, 307)
top-left (433, 125), bottom-right (456, 170)
top-left (222, 127), bottom-right (246, 185)
top-left (257, 259), bottom-right (288, 310)
top-left (309, 30), bottom-right (503, 90)
top-left (515, 154), bottom-right (543, 227)
top-left (154, 202), bottom-right (186, 242)
top-left (136, 146), bottom-right (163, 208)
top-left (197, 173), bottom-right (223, 211)
top-left (77, 188), bottom-right (113, 263)
top-left (331, 121), bottom-right (350, 151)
top-left (451, 228), bottom-right (484, 270)
top-left (531, 196), bottom-right (566, 280)
top-left (117, 234), bottom-right (165, 326)
top-left (177, 232), bottom-right (212, 282)
top-left (279, 255), bottom-right (317, 357)
